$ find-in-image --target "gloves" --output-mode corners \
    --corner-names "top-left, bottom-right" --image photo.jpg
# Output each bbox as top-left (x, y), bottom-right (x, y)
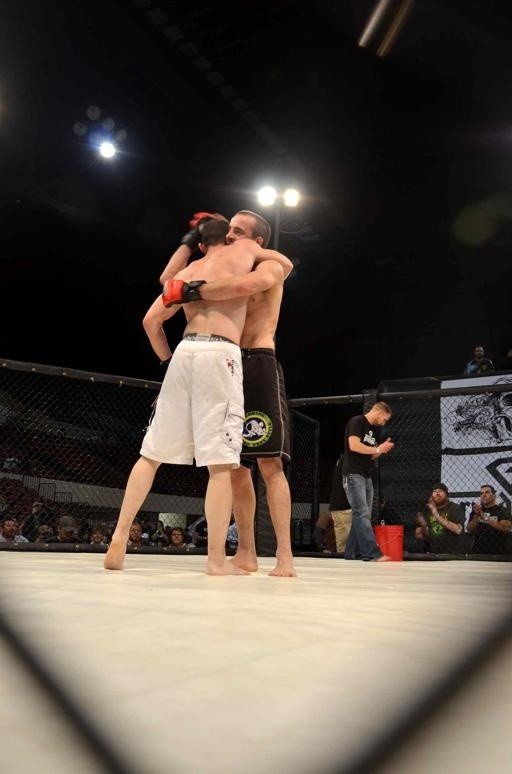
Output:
top-left (180, 212), bottom-right (230, 254)
top-left (161, 278), bottom-right (207, 309)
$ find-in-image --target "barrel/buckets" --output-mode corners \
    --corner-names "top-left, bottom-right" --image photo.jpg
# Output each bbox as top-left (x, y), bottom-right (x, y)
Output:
top-left (374, 525), bottom-right (404, 561)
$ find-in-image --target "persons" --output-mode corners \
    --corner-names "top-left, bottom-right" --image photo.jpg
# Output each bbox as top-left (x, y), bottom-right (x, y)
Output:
top-left (313, 513), bottom-right (337, 553)
top-left (466, 485), bottom-right (510, 554)
top-left (414, 494), bottom-right (434, 541)
top-left (1, 500), bottom-right (238, 547)
top-left (160, 207), bottom-right (296, 579)
top-left (415, 482), bottom-right (465, 553)
top-left (101, 218), bottom-right (293, 575)
top-left (465, 345), bottom-right (495, 378)
top-left (339, 401), bottom-right (397, 562)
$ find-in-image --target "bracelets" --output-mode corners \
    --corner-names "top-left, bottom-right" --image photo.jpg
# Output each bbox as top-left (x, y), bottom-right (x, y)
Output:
top-left (376, 447), bottom-right (379, 456)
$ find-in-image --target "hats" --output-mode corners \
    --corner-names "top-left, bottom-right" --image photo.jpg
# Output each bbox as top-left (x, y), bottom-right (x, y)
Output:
top-left (431, 483), bottom-right (450, 496)
top-left (58, 515), bottom-right (80, 533)
top-left (38, 524), bottom-right (54, 536)
